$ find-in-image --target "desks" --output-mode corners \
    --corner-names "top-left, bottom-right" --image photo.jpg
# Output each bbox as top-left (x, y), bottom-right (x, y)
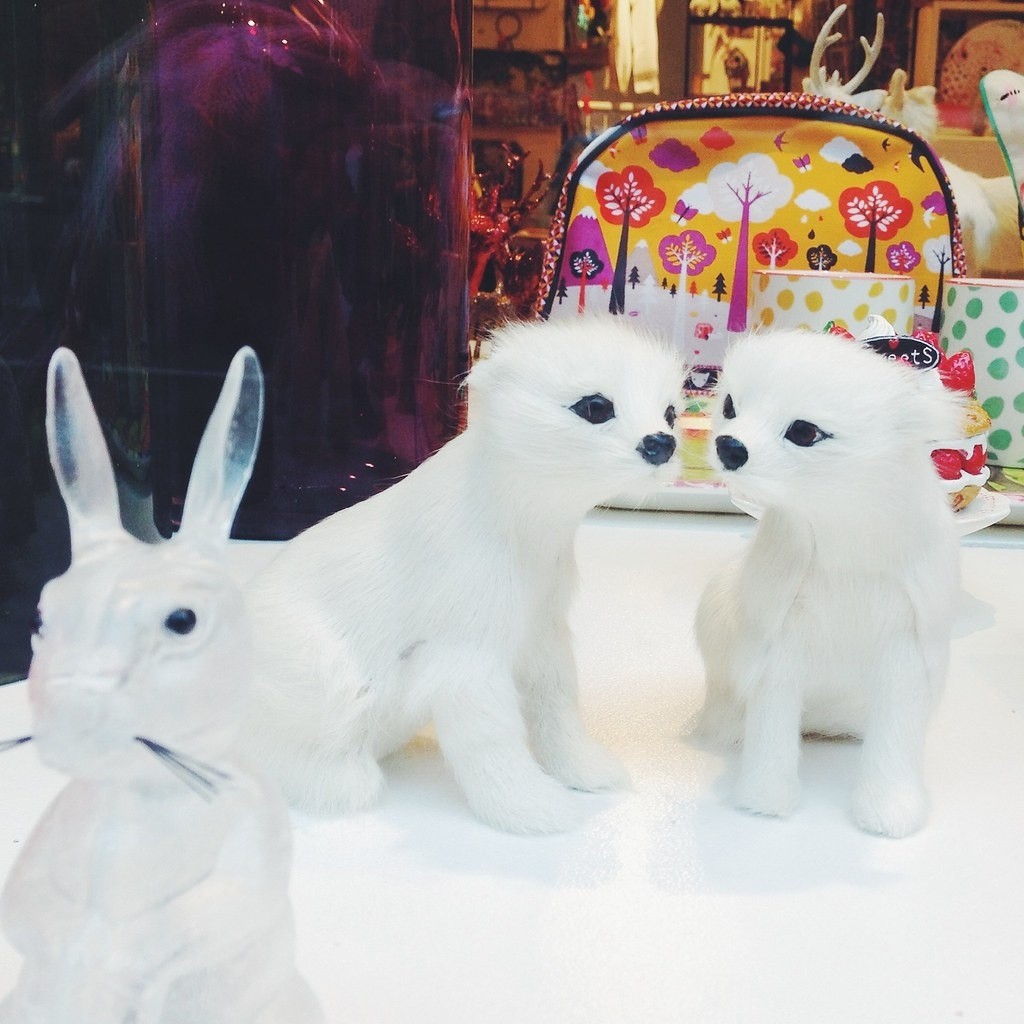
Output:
top-left (0, 483), bottom-right (1024, 1024)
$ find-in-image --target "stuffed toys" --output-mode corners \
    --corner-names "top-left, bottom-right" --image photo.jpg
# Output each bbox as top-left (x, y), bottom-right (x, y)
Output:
top-left (0, 304), bottom-right (973, 1024)
top-left (802, 5), bottom-right (1024, 277)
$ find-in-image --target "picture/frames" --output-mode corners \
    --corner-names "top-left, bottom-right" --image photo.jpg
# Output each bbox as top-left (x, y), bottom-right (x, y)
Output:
top-left (683, 17), bottom-right (793, 99)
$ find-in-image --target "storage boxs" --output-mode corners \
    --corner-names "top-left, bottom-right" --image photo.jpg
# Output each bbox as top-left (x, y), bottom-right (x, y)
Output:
top-left (936, 132), bottom-right (1024, 274)
